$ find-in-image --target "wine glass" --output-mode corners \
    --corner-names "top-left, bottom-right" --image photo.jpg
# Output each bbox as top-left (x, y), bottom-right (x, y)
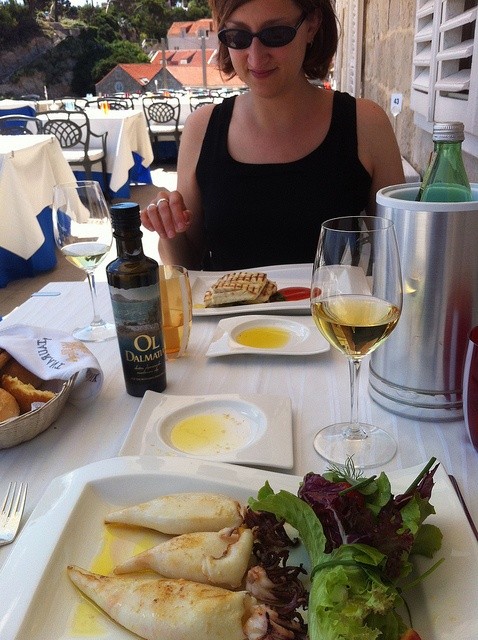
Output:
top-left (52, 180), bottom-right (117, 342)
top-left (310, 215), bottom-right (404, 468)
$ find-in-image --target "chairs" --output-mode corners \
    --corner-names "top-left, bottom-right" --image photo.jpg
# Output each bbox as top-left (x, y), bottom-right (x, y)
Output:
top-left (141, 96), bottom-right (182, 167)
top-left (30, 110), bottom-right (111, 205)
top-left (112, 85), bottom-right (248, 96)
top-left (189, 95), bottom-right (225, 113)
top-left (96, 97), bottom-right (133, 110)
top-left (19, 95), bottom-right (40, 110)
top-left (52, 97), bottom-right (87, 111)
top-left (0, 114), bottom-right (42, 135)
top-left (84, 100), bottom-right (128, 110)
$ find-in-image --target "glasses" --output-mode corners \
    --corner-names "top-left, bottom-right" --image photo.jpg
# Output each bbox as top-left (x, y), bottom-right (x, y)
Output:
top-left (217, 13), bottom-right (309, 47)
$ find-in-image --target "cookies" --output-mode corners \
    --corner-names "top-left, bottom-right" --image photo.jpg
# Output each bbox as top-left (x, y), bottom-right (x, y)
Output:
top-left (0, 348), bottom-right (54, 421)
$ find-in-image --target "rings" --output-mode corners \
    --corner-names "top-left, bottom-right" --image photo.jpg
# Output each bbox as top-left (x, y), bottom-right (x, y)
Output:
top-left (156, 197), bottom-right (167, 206)
top-left (146, 204), bottom-right (156, 210)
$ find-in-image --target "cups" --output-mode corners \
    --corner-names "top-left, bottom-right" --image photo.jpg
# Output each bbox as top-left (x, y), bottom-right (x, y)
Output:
top-left (461, 326), bottom-right (478, 454)
top-left (160, 264), bottom-right (193, 360)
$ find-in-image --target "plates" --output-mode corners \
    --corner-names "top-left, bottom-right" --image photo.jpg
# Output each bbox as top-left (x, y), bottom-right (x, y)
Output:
top-left (0, 455), bottom-right (478, 640)
top-left (117, 389), bottom-right (295, 471)
top-left (205, 314), bottom-right (331, 359)
top-left (189, 263), bottom-right (313, 316)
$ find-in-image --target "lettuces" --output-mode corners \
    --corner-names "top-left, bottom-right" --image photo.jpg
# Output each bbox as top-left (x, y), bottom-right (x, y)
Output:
top-left (248, 464), bottom-right (447, 640)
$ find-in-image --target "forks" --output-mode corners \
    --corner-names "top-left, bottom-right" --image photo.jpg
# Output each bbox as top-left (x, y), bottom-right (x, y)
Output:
top-left (0, 481), bottom-right (28, 546)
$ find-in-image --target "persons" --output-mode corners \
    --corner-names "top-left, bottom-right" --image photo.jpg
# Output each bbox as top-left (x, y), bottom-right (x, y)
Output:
top-left (138, 1), bottom-right (406, 272)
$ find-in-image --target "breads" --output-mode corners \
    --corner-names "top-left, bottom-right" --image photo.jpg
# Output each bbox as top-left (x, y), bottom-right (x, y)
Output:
top-left (203, 272), bottom-right (268, 307)
top-left (242, 280), bottom-right (277, 303)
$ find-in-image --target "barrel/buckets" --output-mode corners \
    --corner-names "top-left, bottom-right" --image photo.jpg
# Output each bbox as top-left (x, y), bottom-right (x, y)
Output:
top-left (368, 184), bottom-right (478, 420)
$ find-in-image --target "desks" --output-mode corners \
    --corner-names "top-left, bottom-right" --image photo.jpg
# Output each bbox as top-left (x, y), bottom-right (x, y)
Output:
top-left (0, 100), bottom-right (35, 116)
top-left (35, 109), bottom-right (146, 190)
top-left (87, 96), bottom-right (225, 158)
top-left (0, 134), bottom-right (59, 278)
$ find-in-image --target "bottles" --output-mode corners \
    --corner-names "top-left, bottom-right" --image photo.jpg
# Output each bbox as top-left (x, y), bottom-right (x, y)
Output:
top-left (106, 202), bottom-right (167, 398)
top-left (415, 121), bottom-right (473, 202)
